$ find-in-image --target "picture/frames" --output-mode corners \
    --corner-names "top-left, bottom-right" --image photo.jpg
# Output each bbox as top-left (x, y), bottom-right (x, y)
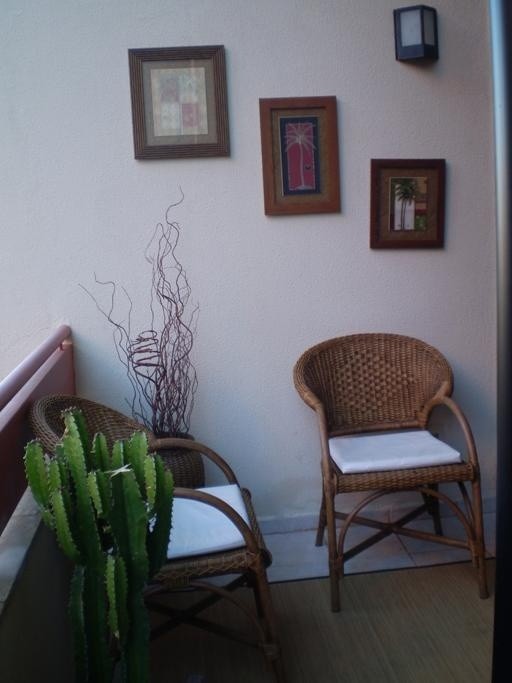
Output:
top-left (125, 43), bottom-right (447, 255)
top-left (367, 156), bottom-right (445, 253)
top-left (256, 94), bottom-right (342, 214)
top-left (128, 45), bottom-right (229, 163)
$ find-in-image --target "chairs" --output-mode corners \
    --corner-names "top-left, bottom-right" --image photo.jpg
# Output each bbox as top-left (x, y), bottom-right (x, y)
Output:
top-left (29, 392), bottom-right (289, 683)
top-left (291, 329), bottom-right (493, 617)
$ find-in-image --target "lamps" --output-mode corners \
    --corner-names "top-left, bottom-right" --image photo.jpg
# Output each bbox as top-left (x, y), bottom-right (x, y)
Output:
top-left (392, 5), bottom-right (439, 66)
